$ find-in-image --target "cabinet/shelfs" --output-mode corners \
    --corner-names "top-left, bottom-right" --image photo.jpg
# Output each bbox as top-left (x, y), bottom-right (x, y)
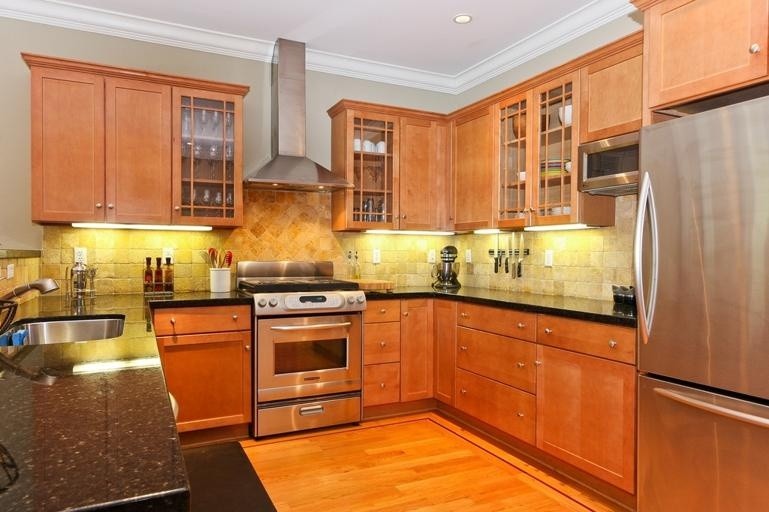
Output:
top-left (173, 76), bottom-right (251, 230)
top-left (448, 95), bottom-right (496, 236)
top-left (326, 98), bottom-right (400, 234)
top-left (148, 298), bottom-right (253, 446)
top-left (434, 293), bottom-right (536, 463)
top-left (496, 45), bottom-right (614, 230)
top-left (629, 0), bottom-right (768, 115)
top-left (363, 294), bottom-right (432, 412)
top-left (537, 306), bottom-right (637, 512)
top-left (401, 108), bottom-right (447, 236)
top-left (21, 51), bottom-right (171, 228)
top-left (578, 28), bottom-right (643, 144)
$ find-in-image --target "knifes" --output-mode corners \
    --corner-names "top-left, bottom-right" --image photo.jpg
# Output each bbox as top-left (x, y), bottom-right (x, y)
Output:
top-left (494, 232), bottom-right (524, 278)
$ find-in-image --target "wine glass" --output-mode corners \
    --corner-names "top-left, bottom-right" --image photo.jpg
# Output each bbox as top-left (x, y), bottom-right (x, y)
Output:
top-left (183, 107), bottom-right (232, 138)
top-left (181, 142), bottom-right (232, 180)
top-left (187, 189), bottom-right (233, 218)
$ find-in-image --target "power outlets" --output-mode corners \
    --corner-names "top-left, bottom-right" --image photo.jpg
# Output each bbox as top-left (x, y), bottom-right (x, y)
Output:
top-left (73, 247), bottom-right (87, 264)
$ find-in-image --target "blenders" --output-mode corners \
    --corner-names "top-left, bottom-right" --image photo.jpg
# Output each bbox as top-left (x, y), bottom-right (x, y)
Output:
top-left (432, 245), bottom-right (462, 289)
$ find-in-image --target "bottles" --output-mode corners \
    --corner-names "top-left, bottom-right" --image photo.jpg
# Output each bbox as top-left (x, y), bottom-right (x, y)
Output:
top-left (164, 256), bottom-right (173, 291)
top-left (144, 257), bottom-right (153, 292)
top-left (70, 260), bottom-right (90, 300)
top-left (154, 257), bottom-right (164, 290)
top-left (345, 250), bottom-right (361, 278)
top-left (355, 198), bottom-right (386, 222)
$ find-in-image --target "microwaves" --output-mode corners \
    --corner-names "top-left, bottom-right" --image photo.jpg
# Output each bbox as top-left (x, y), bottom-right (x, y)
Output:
top-left (577, 130), bottom-right (641, 198)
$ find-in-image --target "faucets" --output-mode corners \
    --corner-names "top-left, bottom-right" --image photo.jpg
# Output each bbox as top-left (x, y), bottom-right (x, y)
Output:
top-left (0, 277), bottom-right (60, 304)
top-left (0, 351), bottom-right (59, 386)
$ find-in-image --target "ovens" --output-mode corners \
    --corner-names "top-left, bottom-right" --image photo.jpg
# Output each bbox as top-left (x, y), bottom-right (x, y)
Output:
top-left (251, 314), bottom-right (364, 440)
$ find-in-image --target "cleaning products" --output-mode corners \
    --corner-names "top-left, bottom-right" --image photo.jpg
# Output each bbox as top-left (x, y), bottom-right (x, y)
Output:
top-left (345, 251), bottom-right (353, 279)
top-left (353, 251), bottom-right (361, 279)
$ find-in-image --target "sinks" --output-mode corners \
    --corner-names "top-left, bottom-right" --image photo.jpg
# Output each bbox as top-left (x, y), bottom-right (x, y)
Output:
top-left (11, 314), bottom-right (126, 344)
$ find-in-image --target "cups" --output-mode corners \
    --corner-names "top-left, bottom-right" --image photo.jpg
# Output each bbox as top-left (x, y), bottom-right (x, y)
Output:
top-left (209, 267), bottom-right (232, 293)
top-left (518, 172), bottom-right (525, 181)
top-left (354, 138), bottom-right (384, 153)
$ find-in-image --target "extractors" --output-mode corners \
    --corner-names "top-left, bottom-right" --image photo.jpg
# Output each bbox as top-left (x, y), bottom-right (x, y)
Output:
top-left (242, 38), bottom-right (356, 190)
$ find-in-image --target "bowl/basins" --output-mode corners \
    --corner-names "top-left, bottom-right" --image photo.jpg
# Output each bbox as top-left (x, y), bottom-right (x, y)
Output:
top-left (557, 105), bottom-right (571, 126)
top-left (512, 115), bottom-right (551, 139)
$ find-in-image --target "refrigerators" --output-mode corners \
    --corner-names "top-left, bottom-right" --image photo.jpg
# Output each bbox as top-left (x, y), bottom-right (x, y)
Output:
top-left (632, 93), bottom-right (769, 510)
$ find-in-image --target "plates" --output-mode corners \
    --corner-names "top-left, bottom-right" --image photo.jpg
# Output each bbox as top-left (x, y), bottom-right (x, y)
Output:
top-left (551, 207), bottom-right (569, 216)
top-left (539, 159), bottom-right (571, 177)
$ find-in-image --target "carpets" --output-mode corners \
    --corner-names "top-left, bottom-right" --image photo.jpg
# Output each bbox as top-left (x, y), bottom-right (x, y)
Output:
top-left (181, 436), bottom-right (279, 511)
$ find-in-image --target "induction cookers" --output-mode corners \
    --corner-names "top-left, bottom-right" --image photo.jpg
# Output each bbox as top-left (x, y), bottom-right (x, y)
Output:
top-left (238, 275), bottom-right (368, 316)
